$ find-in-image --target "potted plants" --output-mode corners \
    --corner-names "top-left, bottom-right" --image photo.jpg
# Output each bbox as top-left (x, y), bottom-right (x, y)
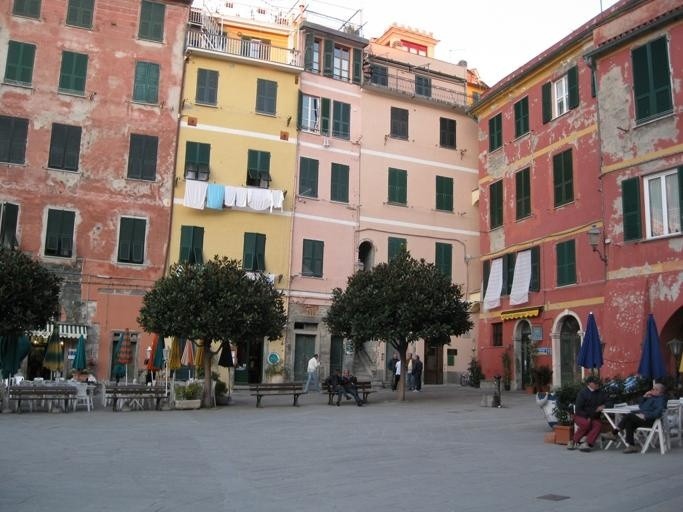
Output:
top-left (552, 385), bottom-right (579, 445)
top-left (523, 342), bottom-right (553, 395)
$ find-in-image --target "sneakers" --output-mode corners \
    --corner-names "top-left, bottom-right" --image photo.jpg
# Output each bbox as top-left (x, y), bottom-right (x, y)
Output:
top-left (566, 440), bottom-right (577, 450)
top-left (600, 431), bottom-right (618, 441)
top-left (623, 444), bottom-right (636, 453)
top-left (580, 443), bottom-right (591, 452)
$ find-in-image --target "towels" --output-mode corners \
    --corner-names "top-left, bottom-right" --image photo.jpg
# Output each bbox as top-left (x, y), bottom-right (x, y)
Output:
top-left (182, 179), bottom-right (246, 211)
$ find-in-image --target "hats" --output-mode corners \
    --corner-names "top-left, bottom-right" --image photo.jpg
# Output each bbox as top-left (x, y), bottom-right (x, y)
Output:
top-left (587, 376), bottom-right (600, 384)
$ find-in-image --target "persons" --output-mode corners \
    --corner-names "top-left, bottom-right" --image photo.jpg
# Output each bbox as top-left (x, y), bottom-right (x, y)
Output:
top-left (599, 382), bottom-right (667, 454)
top-left (301, 353), bottom-right (322, 393)
top-left (404, 352), bottom-right (416, 391)
top-left (342, 368), bottom-right (365, 406)
top-left (387, 351), bottom-right (399, 390)
top-left (291, 4), bottom-right (308, 24)
top-left (390, 359), bottom-right (401, 392)
top-left (565, 375), bottom-right (613, 451)
top-left (411, 354), bottom-right (423, 392)
top-left (324, 367), bottom-right (352, 406)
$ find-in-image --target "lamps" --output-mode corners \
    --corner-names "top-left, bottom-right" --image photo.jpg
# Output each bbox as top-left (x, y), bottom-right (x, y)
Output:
top-left (586, 224), bottom-right (606, 262)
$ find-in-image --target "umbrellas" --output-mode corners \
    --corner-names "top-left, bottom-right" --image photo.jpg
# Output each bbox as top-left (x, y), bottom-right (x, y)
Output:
top-left (71, 333), bottom-right (86, 372)
top-left (144, 334), bottom-right (234, 396)
top-left (41, 318), bottom-right (64, 380)
top-left (0, 335), bottom-right (30, 388)
top-left (573, 310), bottom-right (604, 378)
top-left (117, 327), bottom-right (133, 386)
top-left (107, 331), bottom-right (125, 384)
top-left (635, 312), bottom-right (666, 388)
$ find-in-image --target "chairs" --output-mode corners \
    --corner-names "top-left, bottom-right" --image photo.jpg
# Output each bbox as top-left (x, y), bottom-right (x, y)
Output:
top-left (573, 397), bottom-right (683, 455)
top-left (0, 374), bottom-right (218, 412)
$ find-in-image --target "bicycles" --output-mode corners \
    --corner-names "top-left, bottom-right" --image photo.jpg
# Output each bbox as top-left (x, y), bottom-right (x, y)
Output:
top-left (459, 368), bottom-right (474, 387)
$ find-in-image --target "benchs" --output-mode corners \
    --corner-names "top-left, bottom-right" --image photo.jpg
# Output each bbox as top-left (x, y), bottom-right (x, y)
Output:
top-left (249, 382), bottom-right (308, 408)
top-left (320, 381), bottom-right (378, 405)
